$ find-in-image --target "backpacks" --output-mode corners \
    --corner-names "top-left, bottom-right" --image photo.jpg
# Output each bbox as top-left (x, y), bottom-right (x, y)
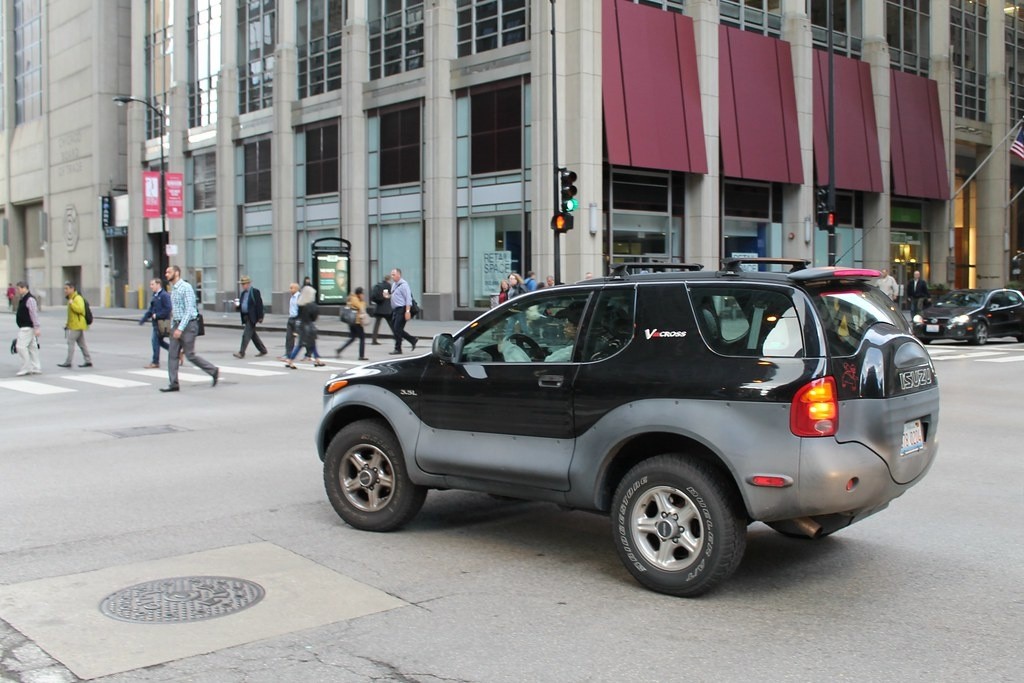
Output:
top-left (409, 299), bottom-right (419, 318)
top-left (73, 293), bottom-right (92, 324)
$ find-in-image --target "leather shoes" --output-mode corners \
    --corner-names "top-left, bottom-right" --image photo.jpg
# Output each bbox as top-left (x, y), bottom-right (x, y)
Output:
top-left (78, 362), bottom-right (92, 367)
top-left (159, 385), bottom-right (179, 391)
top-left (212, 366), bottom-right (218, 386)
top-left (57, 363), bottom-right (71, 367)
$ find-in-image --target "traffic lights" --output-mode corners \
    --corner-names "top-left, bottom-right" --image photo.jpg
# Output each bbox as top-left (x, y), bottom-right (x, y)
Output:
top-left (562, 169), bottom-right (577, 212)
top-left (819, 210), bottom-right (835, 229)
top-left (554, 214), bottom-right (566, 230)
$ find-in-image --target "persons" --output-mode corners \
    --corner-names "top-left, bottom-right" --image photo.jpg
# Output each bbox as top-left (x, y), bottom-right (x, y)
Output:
top-left (138, 277), bottom-right (184, 369)
top-left (907, 270), bottom-right (932, 324)
top-left (159, 265), bottom-right (219, 392)
top-left (585, 272), bottom-right (593, 281)
top-left (543, 301), bottom-right (586, 363)
top-left (232, 267), bottom-right (556, 369)
top-left (57, 282), bottom-right (93, 367)
top-left (15, 281), bottom-right (42, 375)
top-left (6, 283), bottom-right (16, 307)
top-left (875, 269), bottom-right (898, 301)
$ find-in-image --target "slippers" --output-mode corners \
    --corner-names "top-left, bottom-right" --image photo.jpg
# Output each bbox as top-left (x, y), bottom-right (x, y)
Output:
top-left (314, 362), bottom-right (325, 366)
top-left (285, 364), bottom-right (297, 368)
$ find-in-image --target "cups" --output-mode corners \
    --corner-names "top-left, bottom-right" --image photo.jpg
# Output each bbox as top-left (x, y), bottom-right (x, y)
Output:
top-left (383, 289), bottom-right (388, 298)
top-left (234, 299), bottom-right (240, 306)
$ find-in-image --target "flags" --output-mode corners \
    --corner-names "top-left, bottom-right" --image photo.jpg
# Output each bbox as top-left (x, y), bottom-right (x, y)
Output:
top-left (1009, 126), bottom-right (1024, 160)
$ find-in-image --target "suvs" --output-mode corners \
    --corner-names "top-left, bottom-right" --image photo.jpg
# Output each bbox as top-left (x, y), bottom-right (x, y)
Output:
top-left (314, 255), bottom-right (943, 596)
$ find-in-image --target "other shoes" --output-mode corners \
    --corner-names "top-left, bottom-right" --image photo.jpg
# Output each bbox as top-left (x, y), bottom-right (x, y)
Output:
top-left (255, 351), bottom-right (268, 356)
top-left (411, 337), bottom-right (417, 351)
top-left (297, 356), bottom-right (312, 362)
top-left (276, 354), bottom-right (291, 361)
top-left (388, 349), bottom-right (402, 353)
top-left (15, 369), bottom-right (41, 375)
top-left (143, 362), bottom-right (159, 368)
top-left (178, 348), bottom-right (183, 365)
top-left (335, 348), bottom-right (341, 359)
top-left (371, 341), bottom-right (380, 344)
top-left (233, 352), bottom-right (243, 358)
top-left (357, 357), bottom-right (368, 359)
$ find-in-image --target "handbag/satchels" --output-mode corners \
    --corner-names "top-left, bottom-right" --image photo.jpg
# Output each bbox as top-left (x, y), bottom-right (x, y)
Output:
top-left (339, 306), bottom-right (357, 323)
top-left (196, 313), bottom-right (204, 334)
top-left (157, 319), bottom-right (170, 336)
top-left (371, 282), bottom-right (386, 304)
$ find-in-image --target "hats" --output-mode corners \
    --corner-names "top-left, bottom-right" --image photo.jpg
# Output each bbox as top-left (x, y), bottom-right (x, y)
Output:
top-left (236, 274), bottom-right (252, 283)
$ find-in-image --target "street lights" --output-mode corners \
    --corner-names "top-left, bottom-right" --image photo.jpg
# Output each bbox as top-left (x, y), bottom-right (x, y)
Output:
top-left (113, 92), bottom-right (171, 292)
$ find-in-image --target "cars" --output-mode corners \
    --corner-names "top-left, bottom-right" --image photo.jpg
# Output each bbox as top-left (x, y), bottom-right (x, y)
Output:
top-left (911, 285), bottom-right (1024, 346)
